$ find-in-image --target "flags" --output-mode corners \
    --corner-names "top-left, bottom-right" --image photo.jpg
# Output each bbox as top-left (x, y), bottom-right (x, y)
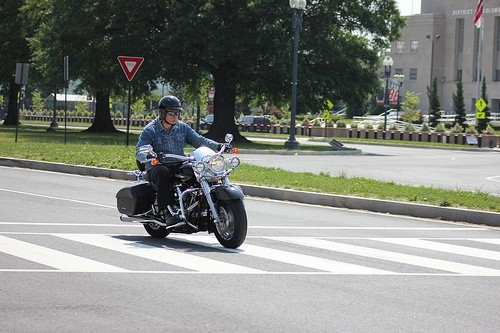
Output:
top-left (473, 0), bottom-right (484, 28)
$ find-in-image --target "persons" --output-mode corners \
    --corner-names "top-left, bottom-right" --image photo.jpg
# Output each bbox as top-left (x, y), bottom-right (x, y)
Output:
top-left (136, 96), bottom-right (234, 225)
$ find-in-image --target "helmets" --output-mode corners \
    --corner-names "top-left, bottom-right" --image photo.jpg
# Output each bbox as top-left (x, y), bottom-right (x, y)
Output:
top-left (158, 95), bottom-right (182, 110)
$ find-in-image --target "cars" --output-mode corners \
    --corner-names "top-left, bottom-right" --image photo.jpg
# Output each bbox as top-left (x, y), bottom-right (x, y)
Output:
top-left (200, 115), bottom-right (213, 126)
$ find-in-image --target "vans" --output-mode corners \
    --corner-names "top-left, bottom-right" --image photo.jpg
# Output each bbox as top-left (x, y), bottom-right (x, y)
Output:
top-left (240, 116), bottom-right (271, 127)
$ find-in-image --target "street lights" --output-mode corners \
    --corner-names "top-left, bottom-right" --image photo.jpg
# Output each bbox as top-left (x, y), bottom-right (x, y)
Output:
top-left (382, 56), bottom-right (394, 131)
top-left (426, 34), bottom-right (440, 124)
top-left (284, 0), bottom-right (306, 148)
top-left (394, 74), bottom-right (405, 121)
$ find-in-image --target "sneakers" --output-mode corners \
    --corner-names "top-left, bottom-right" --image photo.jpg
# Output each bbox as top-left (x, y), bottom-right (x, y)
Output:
top-left (166, 216), bottom-right (186, 229)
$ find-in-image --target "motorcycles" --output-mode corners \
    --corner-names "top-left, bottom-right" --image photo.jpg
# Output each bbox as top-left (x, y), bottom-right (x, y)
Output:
top-left (115, 132), bottom-right (248, 250)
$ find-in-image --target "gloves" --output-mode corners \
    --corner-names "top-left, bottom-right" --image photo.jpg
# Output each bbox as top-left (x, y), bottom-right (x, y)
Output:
top-left (146, 152), bottom-right (157, 159)
top-left (221, 142), bottom-right (233, 151)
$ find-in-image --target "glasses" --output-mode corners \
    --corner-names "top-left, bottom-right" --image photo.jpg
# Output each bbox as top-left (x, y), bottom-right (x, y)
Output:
top-left (167, 111), bottom-right (179, 116)
